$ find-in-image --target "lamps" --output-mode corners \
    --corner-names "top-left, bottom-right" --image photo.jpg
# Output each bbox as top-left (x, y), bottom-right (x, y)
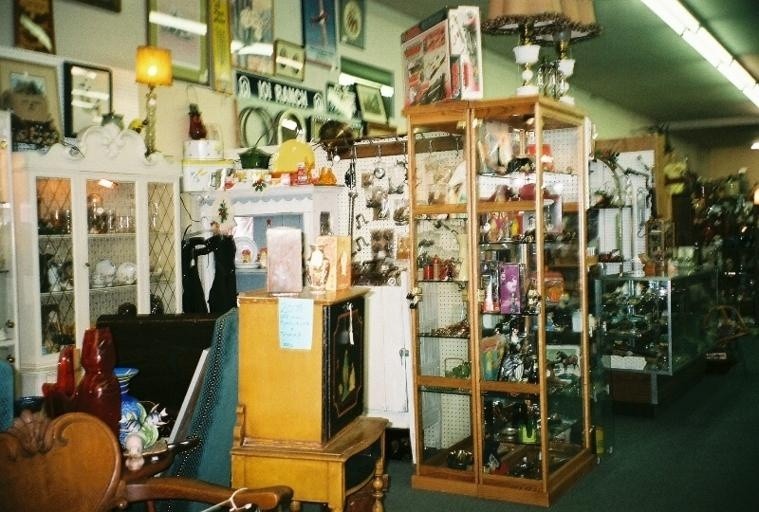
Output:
top-left (480, 1), bottom-right (600, 103)
top-left (136, 41), bottom-right (172, 158)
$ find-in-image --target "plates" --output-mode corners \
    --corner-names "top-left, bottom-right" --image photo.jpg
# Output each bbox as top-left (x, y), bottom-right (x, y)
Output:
top-left (234, 236), bottom-right (261, 268)
top-left (116, 263), bottom-right (138, 285)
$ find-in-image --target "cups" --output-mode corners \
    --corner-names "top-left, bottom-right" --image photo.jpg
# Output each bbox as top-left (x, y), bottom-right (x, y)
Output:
top-left (107, 210), bottom-right (135, 234)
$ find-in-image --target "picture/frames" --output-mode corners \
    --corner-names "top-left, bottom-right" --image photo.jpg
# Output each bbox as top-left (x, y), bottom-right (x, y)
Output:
top-left (355, 80), bottom-right (389, 126)
top-left (62, 60), bottom-right (112, 138)
top-left (274, 36), bottom-right (307, 84)
top-left (12, 1), bottom-right (57, 56)
top-left (148, 0), bottom-right (210, 84)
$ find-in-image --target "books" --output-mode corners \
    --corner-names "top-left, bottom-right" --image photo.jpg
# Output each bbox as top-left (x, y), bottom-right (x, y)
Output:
top-left (318, 233), bottom-right (352, 294)
top-left (265, 226), bottom-right (304, 296)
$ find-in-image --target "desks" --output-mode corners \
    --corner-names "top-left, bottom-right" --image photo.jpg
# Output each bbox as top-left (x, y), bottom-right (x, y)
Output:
top-left (237, 285), bottom-right (370, 450)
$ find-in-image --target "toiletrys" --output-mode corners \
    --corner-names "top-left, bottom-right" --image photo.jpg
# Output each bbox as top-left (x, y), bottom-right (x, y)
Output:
top-left (432, 258), bottom-right (441, 282)
top-left (416, 258), bottom-right (424, 283)
top-left (424, 261), bottom-right (432, 281)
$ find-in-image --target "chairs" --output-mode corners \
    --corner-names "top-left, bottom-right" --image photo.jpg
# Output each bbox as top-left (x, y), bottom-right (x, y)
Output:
top-left (1, 407), bottom-right (295, 509)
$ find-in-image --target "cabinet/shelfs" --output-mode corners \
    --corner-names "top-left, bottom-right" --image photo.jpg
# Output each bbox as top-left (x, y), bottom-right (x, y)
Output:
top-left (4, 110), bottom-right (184, 398)
top-left (366, 287), bottom-right (444, 468)
top-left (401, 95), bottom-right (596, 509)
top-left (230, 416), bottom-right (389, 510)
top-left (584, 135), bottom-right (718, 412)
top-left (227, 182), bottom-right (351, 301)
